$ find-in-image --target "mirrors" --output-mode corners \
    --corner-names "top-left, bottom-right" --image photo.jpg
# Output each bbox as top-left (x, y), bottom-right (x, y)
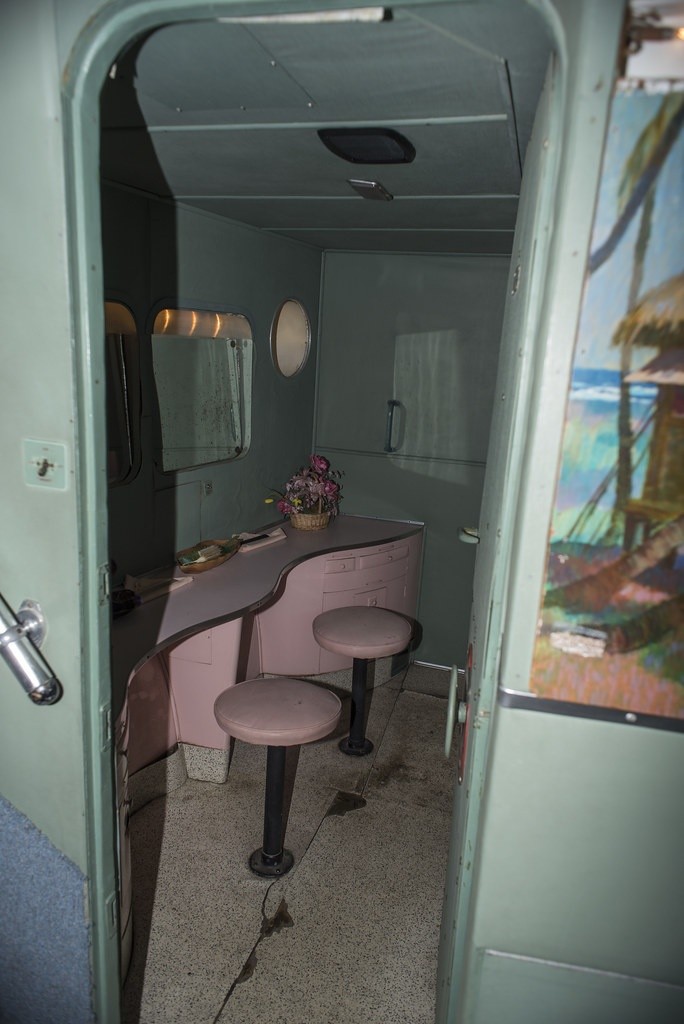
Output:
top-left (102, 300), bottom-right (253, 480)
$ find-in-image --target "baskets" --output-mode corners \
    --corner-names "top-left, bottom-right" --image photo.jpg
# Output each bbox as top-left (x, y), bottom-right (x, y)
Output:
top-left (290, 495), bottom-right (331, 531)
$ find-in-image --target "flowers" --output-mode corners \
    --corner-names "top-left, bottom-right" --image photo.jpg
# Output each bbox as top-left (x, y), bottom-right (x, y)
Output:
top-left (261, 454), bottom-right (344, 522)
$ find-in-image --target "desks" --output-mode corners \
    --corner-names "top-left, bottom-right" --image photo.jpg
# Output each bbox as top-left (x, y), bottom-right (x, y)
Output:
top-left (108, 515), bottom-right (426, 989)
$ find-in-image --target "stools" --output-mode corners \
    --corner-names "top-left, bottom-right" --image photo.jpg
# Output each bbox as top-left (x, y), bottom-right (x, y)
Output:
top-left (312, 606), bottom-right (412, 758)
top-left (213, 676), bottom-right (343, 880)
